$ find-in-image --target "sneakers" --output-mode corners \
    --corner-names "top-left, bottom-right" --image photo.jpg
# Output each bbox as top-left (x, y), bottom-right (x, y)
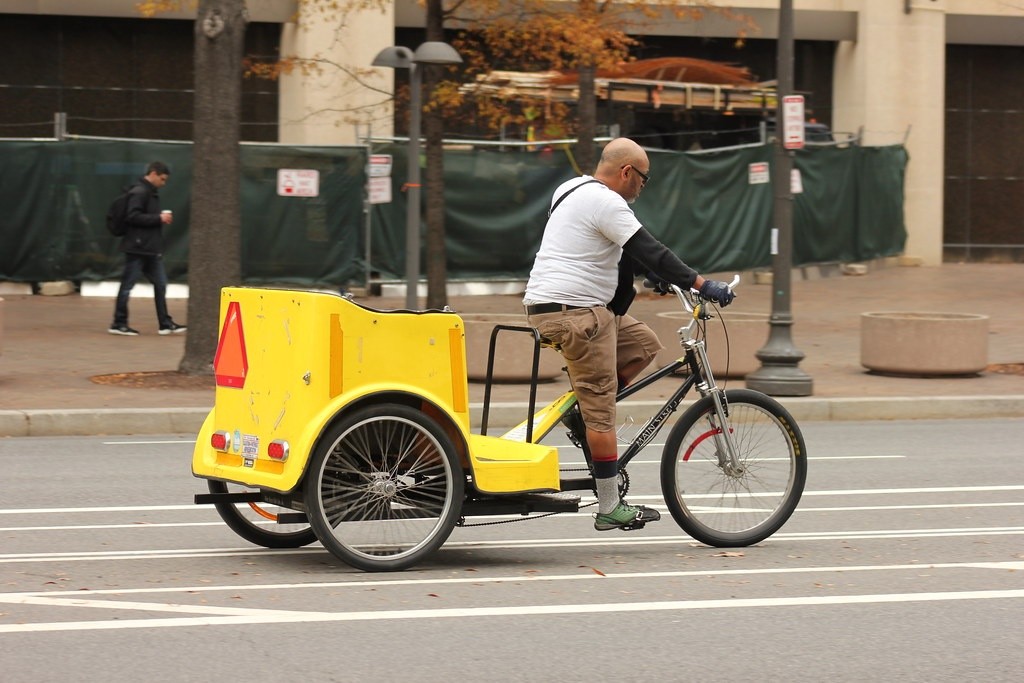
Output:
top-left (158, 321), bottom-right (187, 335)
top-left (562, 407), bottom-right (586, 434)
top-left (108, 324), bottom-right (140, 336)
top-left (592, 494), bottom-right (661, 531)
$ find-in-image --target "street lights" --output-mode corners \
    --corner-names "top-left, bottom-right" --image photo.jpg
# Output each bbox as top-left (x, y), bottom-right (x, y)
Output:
top-left (370, 42), bottom-right (463, 310)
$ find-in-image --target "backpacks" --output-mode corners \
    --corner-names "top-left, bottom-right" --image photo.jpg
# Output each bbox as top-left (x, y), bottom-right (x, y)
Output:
top-left (105, 182), bottom-right (150, 237)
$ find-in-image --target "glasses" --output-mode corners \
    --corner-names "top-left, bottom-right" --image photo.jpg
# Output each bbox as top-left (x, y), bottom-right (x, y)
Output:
top-left (622, 164), bottom-right (651, 184)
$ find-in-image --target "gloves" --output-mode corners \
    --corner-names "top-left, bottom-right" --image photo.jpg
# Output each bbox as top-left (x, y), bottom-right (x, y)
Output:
top-left (699, 280), bottom-right (737, 308)
top-left (651, 276), bottom-right (674, 296)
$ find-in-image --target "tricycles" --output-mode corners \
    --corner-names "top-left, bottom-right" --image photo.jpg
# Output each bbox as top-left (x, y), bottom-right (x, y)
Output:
top-left (191, 275), bottom-right (808, 571)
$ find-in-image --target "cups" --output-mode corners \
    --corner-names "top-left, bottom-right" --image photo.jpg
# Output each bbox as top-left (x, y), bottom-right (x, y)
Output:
top-left (161, 210), bottom-right (172, 225)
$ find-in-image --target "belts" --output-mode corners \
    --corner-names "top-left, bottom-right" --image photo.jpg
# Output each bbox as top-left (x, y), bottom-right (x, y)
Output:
top-left (523, 303), bottom-right (581, 315)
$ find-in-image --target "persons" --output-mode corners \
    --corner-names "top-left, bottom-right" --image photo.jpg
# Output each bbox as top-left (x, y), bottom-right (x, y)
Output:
top-left (108, 163), bottom-right (188, 337)
top-left (521, 137), bottom-right (736, 532)
